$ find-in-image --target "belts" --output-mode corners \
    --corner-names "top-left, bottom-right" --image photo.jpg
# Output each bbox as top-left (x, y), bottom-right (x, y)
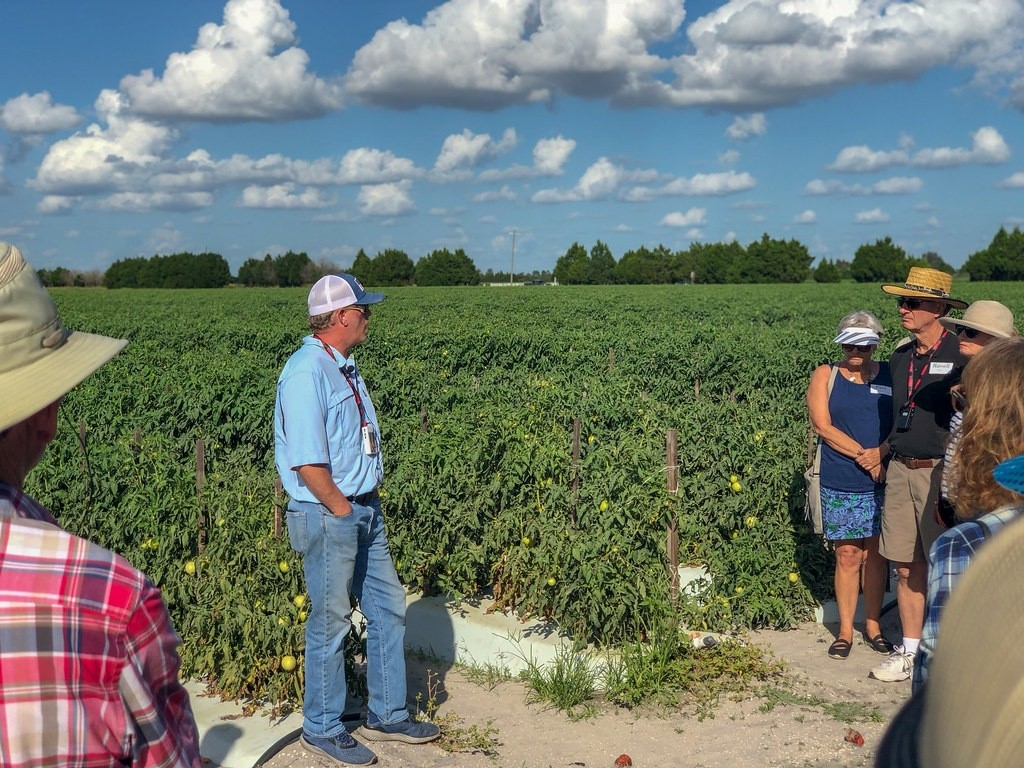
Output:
top-left (348, 486), bottom-right (380, 505)
top-left (892, 454), bottom-right (934, 469)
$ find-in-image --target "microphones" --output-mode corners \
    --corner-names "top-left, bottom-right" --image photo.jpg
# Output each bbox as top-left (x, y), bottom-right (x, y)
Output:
top-left (348, 365), bottom-right (353, 373)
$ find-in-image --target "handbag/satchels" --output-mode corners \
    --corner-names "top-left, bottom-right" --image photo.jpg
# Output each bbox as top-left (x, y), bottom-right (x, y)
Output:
top-left (801, 452), bottom-right (824, 535)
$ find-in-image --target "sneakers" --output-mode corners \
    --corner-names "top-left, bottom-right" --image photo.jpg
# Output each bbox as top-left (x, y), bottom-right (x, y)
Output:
top-left (300, 726), bottom-right (378, 766)
top-left (357, 707), bottom-right (441, 744)
top-left (868, 644), bottom-right (921, 682)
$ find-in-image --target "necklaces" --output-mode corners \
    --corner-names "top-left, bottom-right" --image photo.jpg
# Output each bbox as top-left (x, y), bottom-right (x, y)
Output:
top-left (845, 363), bottom-right (873, 384)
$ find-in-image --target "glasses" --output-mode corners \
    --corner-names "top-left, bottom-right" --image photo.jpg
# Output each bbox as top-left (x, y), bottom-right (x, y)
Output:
top-left (894, 297), bottom-right (927, 310)
top-left (841, 343), bottom-right (871, 352)
top-left (345, 307), bottom-right (373, 318)
top-left (953, 324), bottom-right (980, 338)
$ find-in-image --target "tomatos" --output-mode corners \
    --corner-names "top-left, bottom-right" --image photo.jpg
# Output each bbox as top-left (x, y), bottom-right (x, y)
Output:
top-left (139, 353), bottom-right (799, 671)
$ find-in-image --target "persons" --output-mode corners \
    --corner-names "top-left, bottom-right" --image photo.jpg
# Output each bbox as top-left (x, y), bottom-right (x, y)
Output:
top-left (938, 300), bottom-right (1015, 505)
top-left (870, 267), bottom-right (973, 683)
top-left (1, 239), bottom-right (201, 768)
top-left (910, 335), bottom-right (1024, 691)
top-left (806, 310), bottom-right (895, 659)
top-left (919, 518), bottom-right (1023, 768)
top-left (275, 273), bottom-right (440, 768)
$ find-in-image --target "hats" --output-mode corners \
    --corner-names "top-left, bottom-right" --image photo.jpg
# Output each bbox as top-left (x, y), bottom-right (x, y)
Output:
top-left (0, 240), bottom-right (129, 433)
top-left (308, 274), bottom-right (385, 316)
top-left (939, 299), bottom-right (1016, 340)
top-left (832, 327), bottom-right (879, 345)
top-left (879, 265), bottom-right (969, 310)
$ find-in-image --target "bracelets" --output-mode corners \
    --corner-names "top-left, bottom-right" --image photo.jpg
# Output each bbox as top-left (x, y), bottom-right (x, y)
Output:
top-left (885, 442), bottom-right (893, 455)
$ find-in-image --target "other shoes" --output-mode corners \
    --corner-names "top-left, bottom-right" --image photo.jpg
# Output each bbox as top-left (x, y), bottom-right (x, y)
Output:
top-left (828, 637), bottom-right (853, 660)
top-left (863, 630), bottom-right (894, 656)
